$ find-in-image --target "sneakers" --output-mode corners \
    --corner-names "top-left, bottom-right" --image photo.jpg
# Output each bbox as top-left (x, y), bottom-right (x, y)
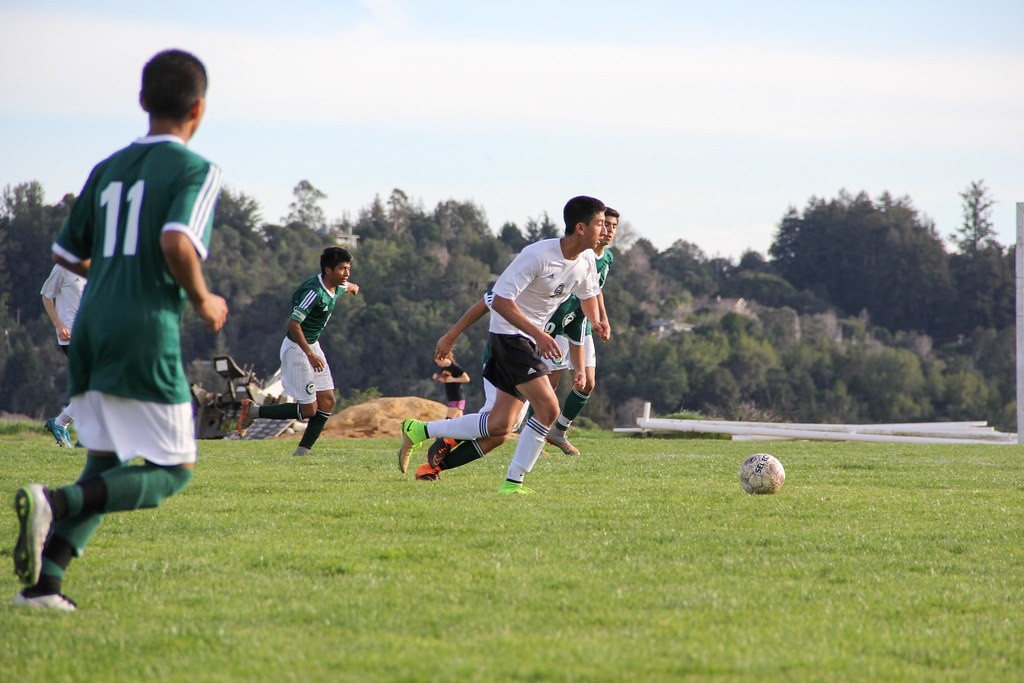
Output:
top-left (293, 446), bottom-right (319, 459)
top-left (538, 450), bottom-right (549, 458)
top-left (398, 419), bottom-right (427, 473)
top-left (415, 463), bottom-right (443, 484)
top-left (15, 586), bottom-right (77, 613)
top-left (12, 483), bottom-right (56, 588)
top-left (497, 480), bottom-right (539, 499)
top-left (428, 437), bottom-right (451, 469)
top-left (545, 425), bottom-right (581, 456)
top-left (45, 418), bottom-right (72, 450)
top-left (238, 399), bottom-right (256, 439)
top-left (74, 439), bottom-right (84, 448)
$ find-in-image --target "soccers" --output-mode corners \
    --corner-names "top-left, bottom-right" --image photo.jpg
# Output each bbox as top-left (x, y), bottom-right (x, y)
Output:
top-left (738, 451), bottom-right (786, 495)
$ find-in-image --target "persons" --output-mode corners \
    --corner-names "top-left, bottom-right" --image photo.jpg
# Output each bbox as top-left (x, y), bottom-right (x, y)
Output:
top-left (398, 195), bottom-right (619, 493)
top-left (235, 246), bottom-right (359, 458)
top-left (12, 46), bottom-right (228, 610)
top-left (41, 260), bottom-right (90, 449)
